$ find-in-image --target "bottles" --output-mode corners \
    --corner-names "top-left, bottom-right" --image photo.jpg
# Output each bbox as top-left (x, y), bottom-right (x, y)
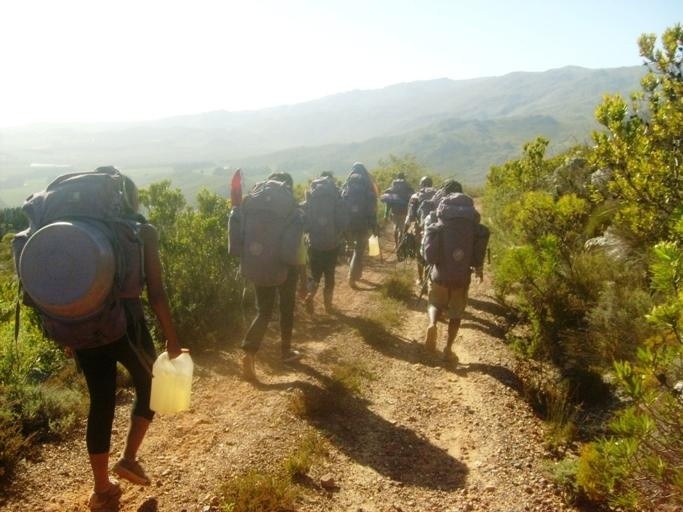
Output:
top-left (228, 205), bottom-right (239, 219)
top-left (423, 211), bottom-right (437, 232)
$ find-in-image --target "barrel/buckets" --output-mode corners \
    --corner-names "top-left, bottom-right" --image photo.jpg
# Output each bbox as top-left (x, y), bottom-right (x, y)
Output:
top-left (369, 233), bottom-right (380, 257)
top-left (149, 348), bottom-right (194, 414)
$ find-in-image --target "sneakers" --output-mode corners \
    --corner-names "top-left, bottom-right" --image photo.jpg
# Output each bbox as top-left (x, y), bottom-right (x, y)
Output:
top-left (303, 294), bottom-right (340, 316)
top-left (346, 271), bottom-right (364, 291)
top-left (87, 478), bottom-right (122, 512)
top-left (423, 324), bottom-right (459, 363)
top-left (240, 351), bottom-right (257, 381)
top-left (280, 350), bottom-right (301, 363)
top-left (110, 455), bottom-right (151, 486)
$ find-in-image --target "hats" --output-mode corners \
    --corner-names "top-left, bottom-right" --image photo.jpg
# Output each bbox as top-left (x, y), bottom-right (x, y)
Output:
top-left (439, 178), bottom-right (463, 196)
top-left (418, 176), bottom-right (432, 187)
top-left (396, 172), bottom-right (408, 178)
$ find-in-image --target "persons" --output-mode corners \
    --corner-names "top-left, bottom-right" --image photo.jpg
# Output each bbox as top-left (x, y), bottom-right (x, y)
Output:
top-left (53, 165), bottom-right (182, 498)
top-left (299, 171), bottom-right (353, 314)
top-left (239, 168), bottom-right (310, 379)
top-left (337, 159), bottom-right (378, 287)
top-left (382, 172), bottom-right (414, 263)
top-left (424, 178), bottom-right (471, 363)
top-left (403, 175), bottom-right (441, 295)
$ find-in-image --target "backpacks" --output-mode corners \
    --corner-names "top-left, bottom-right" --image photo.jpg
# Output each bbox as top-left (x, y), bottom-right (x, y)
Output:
top-left (339, 173), bottom-right (376, 241)
top-left (225, 178), bottom-right (303, 288)
top-left (416, 188), bottom-right (437, 238)
top-left (421, 191), bottom-right (490, 290)
top-left (298, 175), bottom-right (346, 253)
top-left (379, 177), bottom-right (412, 219)
top-left (9, 169), bottom-right (143, 352)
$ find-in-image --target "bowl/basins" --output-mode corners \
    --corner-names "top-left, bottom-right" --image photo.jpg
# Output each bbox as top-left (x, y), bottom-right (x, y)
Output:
top-left (15, 217), bottom-right (127, 322)
